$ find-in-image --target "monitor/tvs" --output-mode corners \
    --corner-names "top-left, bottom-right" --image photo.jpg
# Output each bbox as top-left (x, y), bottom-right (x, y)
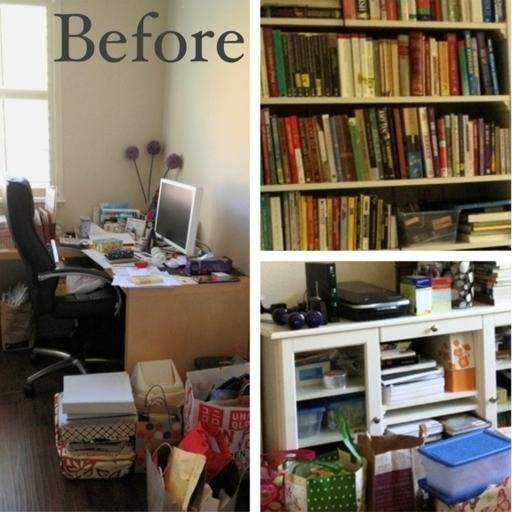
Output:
top-left (154, 178), bottom-right (203, 256)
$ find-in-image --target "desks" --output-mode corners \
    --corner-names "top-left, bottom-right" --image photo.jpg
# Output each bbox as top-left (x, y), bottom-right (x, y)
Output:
top-left (0, 230), bottom-right (246, 382)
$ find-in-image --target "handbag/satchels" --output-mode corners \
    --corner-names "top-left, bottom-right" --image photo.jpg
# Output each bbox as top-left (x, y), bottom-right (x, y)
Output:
top-left (136, 364), bottom-right (428, 512)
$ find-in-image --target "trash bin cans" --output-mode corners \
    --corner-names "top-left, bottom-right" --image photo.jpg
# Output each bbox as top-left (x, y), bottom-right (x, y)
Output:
top-left (130, 359), bottom-right (186, 415)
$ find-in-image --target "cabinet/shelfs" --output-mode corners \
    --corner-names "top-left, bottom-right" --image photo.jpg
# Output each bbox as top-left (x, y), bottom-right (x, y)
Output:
top-left (249, 1), bottom-right (511, 511)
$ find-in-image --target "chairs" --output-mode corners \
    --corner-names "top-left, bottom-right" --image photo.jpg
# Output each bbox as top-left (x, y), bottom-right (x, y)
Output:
top-left (7, 173), bottom-right (123, 397)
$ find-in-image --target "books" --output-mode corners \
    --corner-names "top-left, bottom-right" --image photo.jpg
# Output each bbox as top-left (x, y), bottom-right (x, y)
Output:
top-left (474, 262), bottom-right (512, 305)
top-left (58, 371), bottom-right (137, 451)
top-left (381, 349), bottom-right (492, 439)
top-left (260, 0), bottom-right (511, 250)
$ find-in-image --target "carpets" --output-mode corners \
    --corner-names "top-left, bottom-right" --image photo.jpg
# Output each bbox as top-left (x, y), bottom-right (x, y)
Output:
top-left (11, 340), bottom-right (122, 393)
